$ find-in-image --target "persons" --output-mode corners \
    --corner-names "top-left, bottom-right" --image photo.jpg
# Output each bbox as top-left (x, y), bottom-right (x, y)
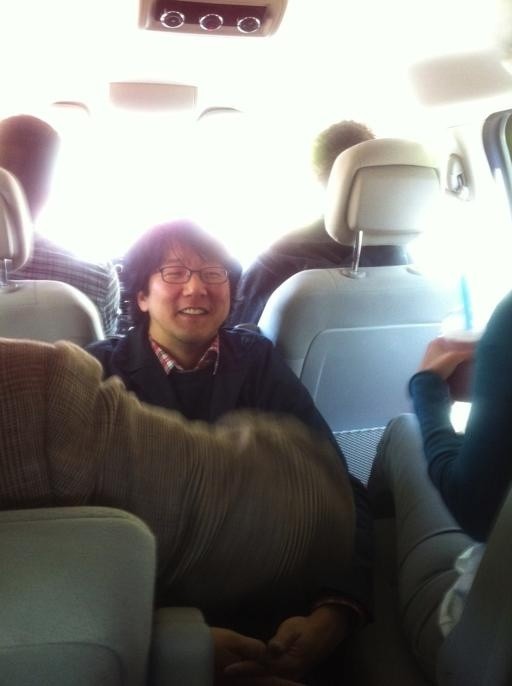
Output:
top-left (0, 113), bottom-right (122, 338)
top-left (226, 117), bottom-right (410, 328)
top-left (363, 289), bottom-right (512, 685)
top-left (78, 218), bottom-right (376, 686)
top-left (0, 334), bottom-right (356, 642)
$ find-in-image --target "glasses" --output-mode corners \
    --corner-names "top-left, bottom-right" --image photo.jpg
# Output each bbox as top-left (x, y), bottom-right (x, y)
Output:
top-left (151, 265), bottom-right (229, 284)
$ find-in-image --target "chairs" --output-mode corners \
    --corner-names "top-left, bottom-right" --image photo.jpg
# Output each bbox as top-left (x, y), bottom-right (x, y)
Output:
top-left (255, 137), bottom-right (466, 490)
top-left (2, 503), bottom-right (215, 686)
top-left (1, 166), bottom-right (107, 349)
